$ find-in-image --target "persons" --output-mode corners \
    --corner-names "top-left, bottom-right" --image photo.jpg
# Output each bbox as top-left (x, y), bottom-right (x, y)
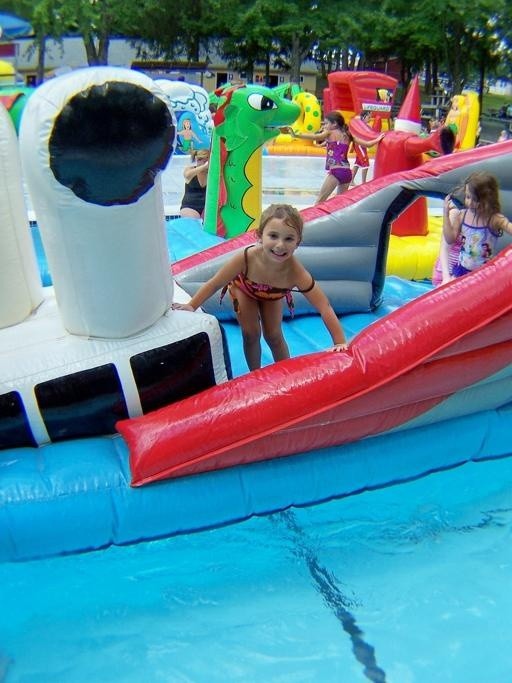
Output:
top-left (497, 129), bottom-right (509, 143)
top-left (350, 110), bottom-right (374, 187)
top-left (427, 114), bottom-right (445, 135)
top-left (177, 117), bottom-right (202, 152)
top-left (179, 149), bottom-right (210, 220)
top-left (169, 201), bottom-right (349, 373)
top-left (443, 167), bottom-right (512, 278)
top-left (429, 186), bottom-right (466, 288)
top-left (287, 110), bottom-right (386, 207)
